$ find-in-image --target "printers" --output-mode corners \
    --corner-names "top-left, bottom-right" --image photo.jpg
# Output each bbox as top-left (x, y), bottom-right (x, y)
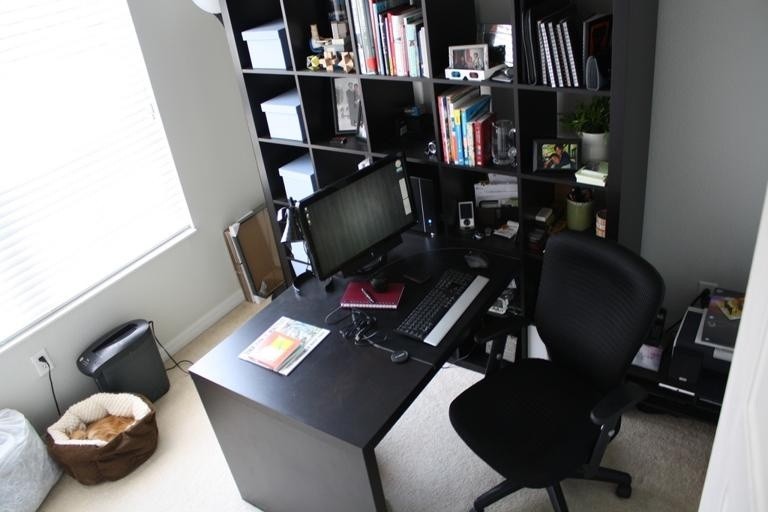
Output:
top-left (657, 306), bottom-right (734, 408)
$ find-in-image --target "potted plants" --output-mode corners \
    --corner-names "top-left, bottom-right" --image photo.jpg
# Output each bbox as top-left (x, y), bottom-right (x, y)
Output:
top-left (557, 95), bottom-right (612, 164)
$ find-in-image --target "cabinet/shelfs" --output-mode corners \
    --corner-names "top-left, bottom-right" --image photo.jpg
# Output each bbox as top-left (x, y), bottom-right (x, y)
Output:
top-left (219, 0), bottom-right (660, 296)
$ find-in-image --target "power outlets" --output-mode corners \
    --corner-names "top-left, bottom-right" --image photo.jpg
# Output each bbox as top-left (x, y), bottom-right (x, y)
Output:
top-left (29, 347), bottom-right (55, 378)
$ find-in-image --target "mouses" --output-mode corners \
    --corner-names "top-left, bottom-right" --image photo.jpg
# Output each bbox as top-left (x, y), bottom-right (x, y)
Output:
top-left (464, 250), bottom-right (490, 269)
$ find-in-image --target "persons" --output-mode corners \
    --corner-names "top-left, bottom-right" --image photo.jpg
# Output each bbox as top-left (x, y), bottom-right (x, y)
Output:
top-left (543, 152), bottom-right (570, 170)
top-left (345, 81), bottom-right (357, 126)
top-left (555, 144), bottom-right (572, 169)
top-left (352, 84), bottom-right (359, 116)
top-left (451, 50), bottom-right (483, 71)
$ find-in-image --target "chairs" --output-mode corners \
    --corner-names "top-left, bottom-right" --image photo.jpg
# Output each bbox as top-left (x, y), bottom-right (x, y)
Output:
top-left (449, 229), bottom-right (666, 510)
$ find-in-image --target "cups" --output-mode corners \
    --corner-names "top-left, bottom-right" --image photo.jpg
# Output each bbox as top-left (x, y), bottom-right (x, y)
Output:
top-left (566, 191), bottom-right (594, 233)
top-left (595, 207), bottom-right (607, 238)
top-left (491, 119), bottom-right (517, 168)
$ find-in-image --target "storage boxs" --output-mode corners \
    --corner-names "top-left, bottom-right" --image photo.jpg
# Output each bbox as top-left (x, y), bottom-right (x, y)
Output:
top-left (278, 152), bottom-right (318, 201)
top-left (260, 88), bottom-right (307, 143)
top-left (241, 19), bottom-right (291, 70)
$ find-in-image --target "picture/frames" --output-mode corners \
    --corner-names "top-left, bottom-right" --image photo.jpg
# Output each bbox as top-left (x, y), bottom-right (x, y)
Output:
top-left (532, 138), bottom-right (581, 174)
top-left (330, 77), bottom-right (361, 137)
top-left (448, 44), bottom-right (489, 72)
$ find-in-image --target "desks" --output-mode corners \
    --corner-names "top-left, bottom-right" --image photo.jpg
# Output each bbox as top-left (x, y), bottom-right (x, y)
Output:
top-left (187, 229), bottom-right (529, 510)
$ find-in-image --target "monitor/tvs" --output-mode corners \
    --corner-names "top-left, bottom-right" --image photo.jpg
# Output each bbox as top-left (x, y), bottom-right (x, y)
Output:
top-left (295, 149), bottom-right (419, 282)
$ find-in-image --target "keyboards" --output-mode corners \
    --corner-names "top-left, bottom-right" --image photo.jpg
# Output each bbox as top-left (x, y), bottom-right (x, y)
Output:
top-left (393, 267), bottom-right (492, 347)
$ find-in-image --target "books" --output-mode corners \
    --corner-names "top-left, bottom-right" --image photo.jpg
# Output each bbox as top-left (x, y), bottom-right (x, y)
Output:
top-left (573, 159), bottom-right (609, 188)
top-left (434, 83), bottom-right (497, 168)
top-left (339, 278), bottom-right (406, 310)
top-left (694, 296), bottom-right (746, 362)
top-left (236, 314), bottom-right (332, 376)
top-left (248, 328), bottom-right (305, 370)
top-left (346, 0), bottom-right (432, 80)
top-left (519, 1), bottom-right (612, 92)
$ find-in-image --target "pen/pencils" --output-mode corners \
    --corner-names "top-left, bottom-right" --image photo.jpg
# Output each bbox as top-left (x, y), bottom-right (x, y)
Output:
top-left (361, 288), bottom-right (375, 304)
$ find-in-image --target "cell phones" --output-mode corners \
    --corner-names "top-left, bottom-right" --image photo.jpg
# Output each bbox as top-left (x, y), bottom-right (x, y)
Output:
top-left (398, 265), bottom-right (433, 284)
